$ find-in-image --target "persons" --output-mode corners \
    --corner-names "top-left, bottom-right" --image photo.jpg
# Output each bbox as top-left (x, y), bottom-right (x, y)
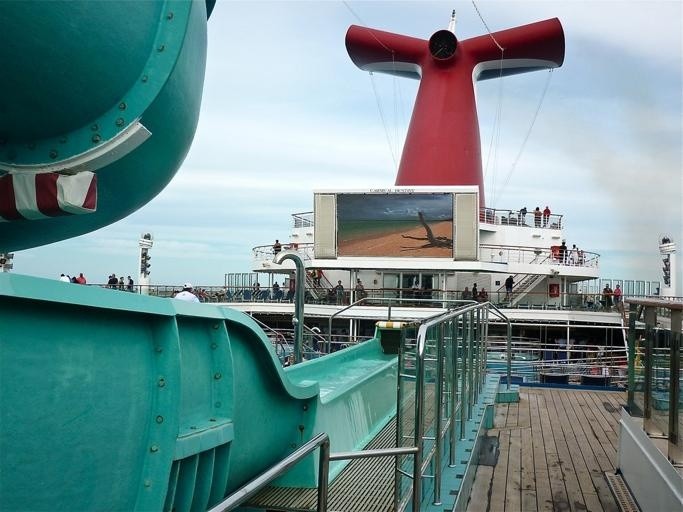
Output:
top-left (461, 286), bottom-right (469, 306)
top-left (310, 327), bottom-right (352, 354)
top-left (613, 284), bottom-right (623, 307)
top-left (104, 274), bottom-right (134, 293)
top-left (539, 332), bottom-right (617, 365)
top-left (179, 278), bottom-right (365, 306)
top-left (284, 351), bottom-right (306, 367)
top-left (476, 287), bottom-right (487, 302)
top-left (273, 240), bottom-right (281, 256)
top-left (517, 208), bottom-right (527, 226)
top-left (471, 282), bottom-right (479, 301)
top-left (600, 284), bottom-right (614, 310)
top-left (59, 273), bottom-right (87, 284)
top-left (542, 206), bottom-right (551, 227)
top-left (559, 240), bottom-right (586, 266)
top-left (534, 208), bottom-right (542, 228)
top-left (505, 276), bottom-right (515, 303)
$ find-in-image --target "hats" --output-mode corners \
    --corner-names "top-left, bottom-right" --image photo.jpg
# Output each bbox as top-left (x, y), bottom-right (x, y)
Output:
top-left (183, 282), bottom-right (193, 289)
